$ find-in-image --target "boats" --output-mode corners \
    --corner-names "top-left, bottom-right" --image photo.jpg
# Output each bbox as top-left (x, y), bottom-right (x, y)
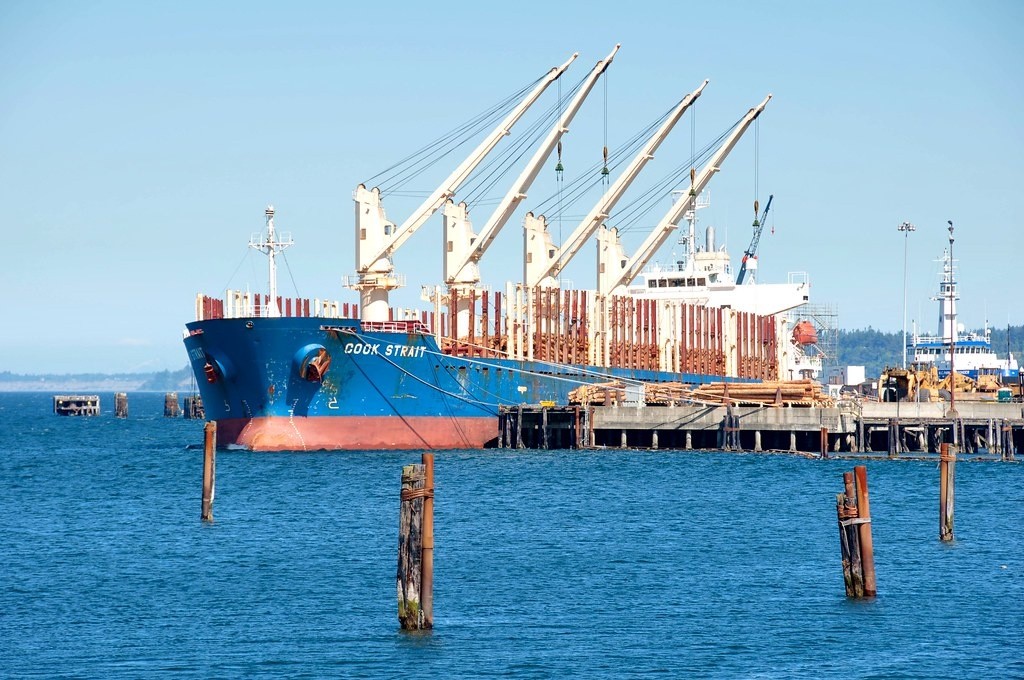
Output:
top-left (178, 40), bottom-right (821, 456)
top-left (903, 216), bottom-right (1021, 389)
